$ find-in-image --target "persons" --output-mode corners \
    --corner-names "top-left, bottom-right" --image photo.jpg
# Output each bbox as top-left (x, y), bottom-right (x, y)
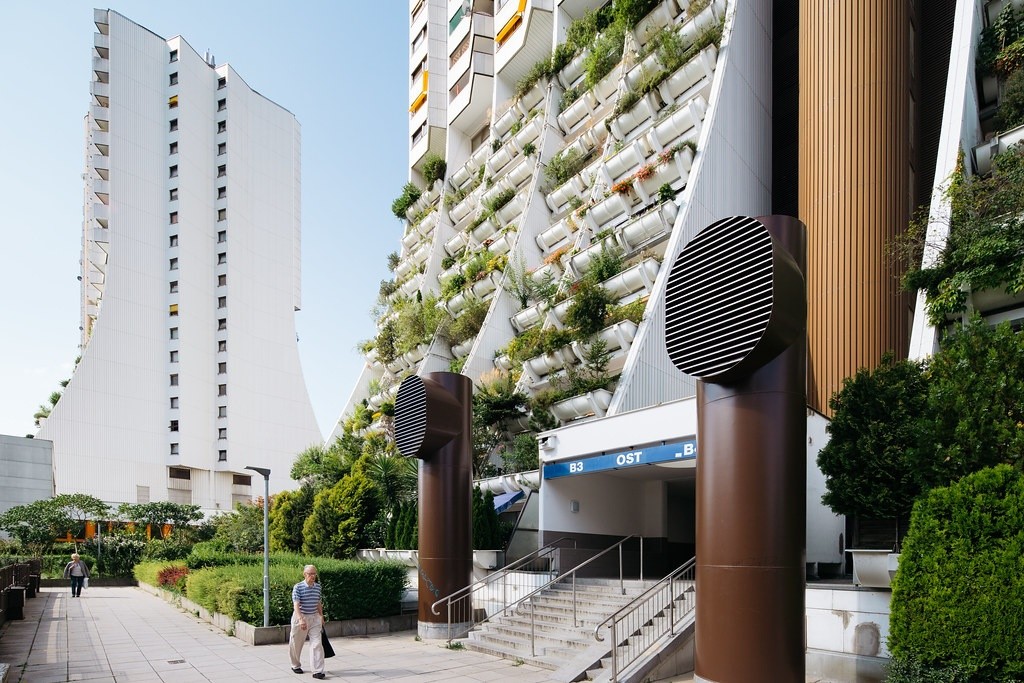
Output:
top-left (289, 565), bottom-right (327, 679)
top-left (67, 553), bottom-right (87, 597)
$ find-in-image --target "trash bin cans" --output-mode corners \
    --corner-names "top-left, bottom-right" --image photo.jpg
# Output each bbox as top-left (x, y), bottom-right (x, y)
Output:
top-left (28, 575), bottom-right (37, 598)
top-left (7, 585), bottom-right (26, 621)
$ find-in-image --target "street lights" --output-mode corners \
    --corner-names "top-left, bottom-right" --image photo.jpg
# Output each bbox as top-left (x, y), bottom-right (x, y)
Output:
top-left (244, 465), bottom-right (271, 627)
top-left (97, 520), bottom-right (106, 560)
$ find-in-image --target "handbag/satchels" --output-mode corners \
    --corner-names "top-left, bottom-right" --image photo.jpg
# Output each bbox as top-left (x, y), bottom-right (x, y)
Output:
top-left (82, 578), bottom-right (89, 589)
top-left (321, 625), bottom-right (335, 658)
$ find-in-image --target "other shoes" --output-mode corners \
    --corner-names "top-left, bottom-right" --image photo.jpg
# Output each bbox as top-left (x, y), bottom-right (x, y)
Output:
top-left (313, 673), bottom-right (325, 679)
top-left (76, 595), bottom-right (79, 597)
top-left (72, 596), bottom-right (75, 597)
top-left (291, 668), bottom-right (303, 674)
